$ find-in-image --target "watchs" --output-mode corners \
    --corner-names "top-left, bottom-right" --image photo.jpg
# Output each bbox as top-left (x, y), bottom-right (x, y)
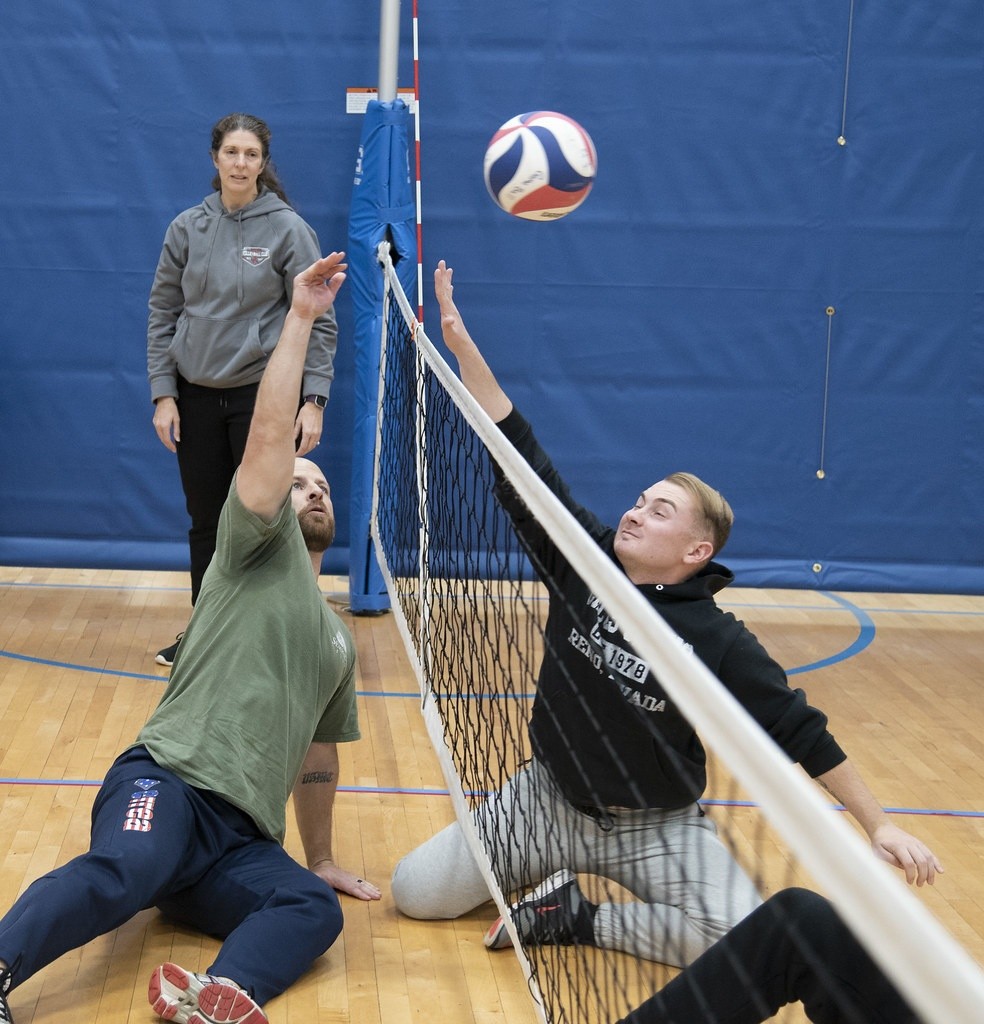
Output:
top-left (304, 394), bottom-right (327, 408)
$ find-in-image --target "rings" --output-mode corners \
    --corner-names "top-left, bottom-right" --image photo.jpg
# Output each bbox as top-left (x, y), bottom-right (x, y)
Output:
top-left (357, 880), bottom-right (362, 883)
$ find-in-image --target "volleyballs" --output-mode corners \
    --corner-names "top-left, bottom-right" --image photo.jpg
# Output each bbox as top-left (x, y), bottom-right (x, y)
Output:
top-left (478, 106), bottom-right (603, 225)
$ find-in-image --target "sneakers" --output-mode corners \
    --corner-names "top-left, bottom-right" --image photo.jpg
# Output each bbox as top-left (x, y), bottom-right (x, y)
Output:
top-left (481, 868), bottom-right (591, 950)
top-left (154, 630), bottom-right (185, 668)
top-left (0, 950), bottom-right (26, 1024)
top-left (147, 961), bottom-right (271, 1024)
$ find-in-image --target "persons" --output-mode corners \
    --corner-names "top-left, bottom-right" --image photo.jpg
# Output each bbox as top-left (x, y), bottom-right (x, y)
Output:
top-left (0, 250), bottom-right (382, 1024)
top-left (391, 261), bottom-right (944, 969)
top-left (147, 113), bottom-right (338, 666)
